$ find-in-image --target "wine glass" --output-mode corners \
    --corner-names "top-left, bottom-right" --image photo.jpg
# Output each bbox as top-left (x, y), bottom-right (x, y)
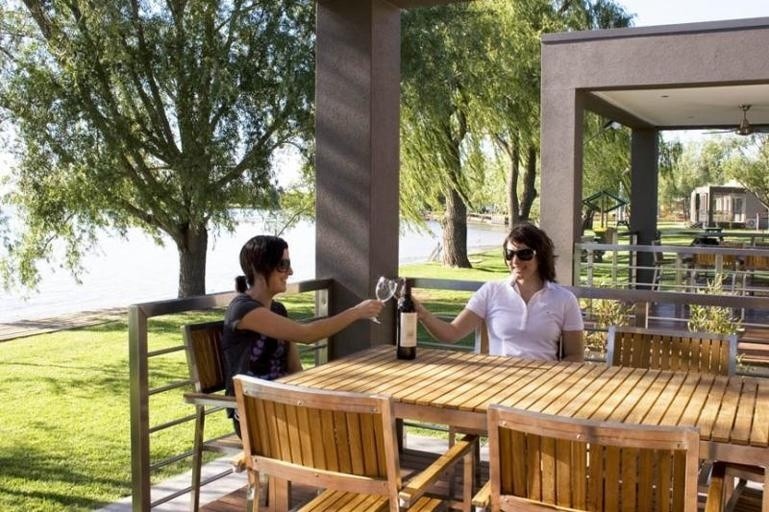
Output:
top-left (368, 276), bottom-right (397, 325)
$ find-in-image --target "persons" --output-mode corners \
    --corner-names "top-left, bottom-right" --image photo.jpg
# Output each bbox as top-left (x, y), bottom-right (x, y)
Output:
top-left (402, 223), bottom-right (587, 365)
top-left (219, 233), bottom-right (384, 449)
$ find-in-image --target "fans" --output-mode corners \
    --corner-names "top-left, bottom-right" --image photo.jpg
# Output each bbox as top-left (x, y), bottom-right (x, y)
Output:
top-left (701, 104), bottom-right (769, 135)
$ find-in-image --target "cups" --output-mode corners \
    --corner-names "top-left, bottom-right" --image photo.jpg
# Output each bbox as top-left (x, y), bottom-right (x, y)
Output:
top-left (388, 278), bottom-right (405, 300)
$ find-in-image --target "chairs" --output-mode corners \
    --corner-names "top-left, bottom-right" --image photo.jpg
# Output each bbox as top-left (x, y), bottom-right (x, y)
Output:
top-left (447, 322), bottom-right (561, 498)
top-left (689, 241), bottom-right (769, 296)
top-left (703, 462), bottom-right (767, 512)
top-left (233, 374), bottom-right (479, 512)
top-left (602, 323), bottom-right (738, 376)
top-left (182, 319), bottom-right (237, 511)
top-left (471, 404), bottom-right (700, 511)
top-left (651, 240), bottom-right (676, 306)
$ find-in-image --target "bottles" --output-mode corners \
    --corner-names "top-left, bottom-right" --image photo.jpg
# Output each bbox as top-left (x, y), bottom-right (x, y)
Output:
top-left (397, 277), bottom-right (417, 360)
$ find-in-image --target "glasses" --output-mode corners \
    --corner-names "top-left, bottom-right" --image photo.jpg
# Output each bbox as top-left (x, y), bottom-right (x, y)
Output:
top-left (506, 248), bottom-right (536, 260)
top-left (276, 259), bottom-right (290, 272)
